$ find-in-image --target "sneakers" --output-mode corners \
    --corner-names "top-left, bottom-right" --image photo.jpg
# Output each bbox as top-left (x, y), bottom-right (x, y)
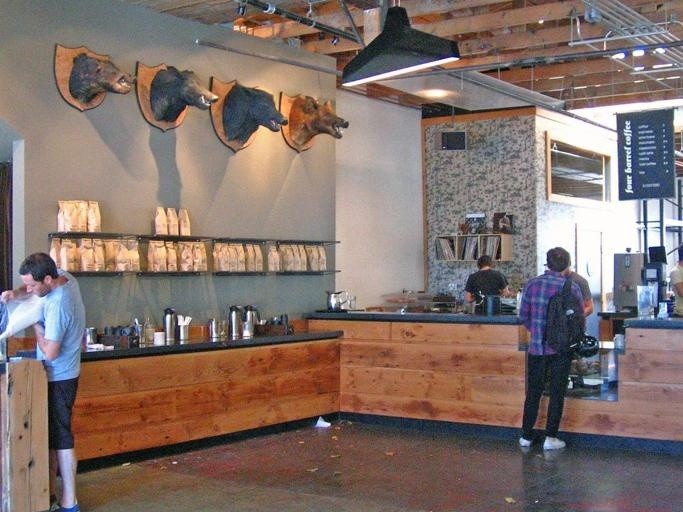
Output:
top-left (46, 500), bottom-right (80, 512)
top-left (544, 437), bottom-right (566, 451)
top-left (519, 436), bottom-right (533, 446)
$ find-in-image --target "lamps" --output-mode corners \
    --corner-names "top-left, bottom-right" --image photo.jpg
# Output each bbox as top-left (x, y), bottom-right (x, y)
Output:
top-left (339, 2), bottom-right (464, 91)
top-left (234, 1), bottom-right (340, 50)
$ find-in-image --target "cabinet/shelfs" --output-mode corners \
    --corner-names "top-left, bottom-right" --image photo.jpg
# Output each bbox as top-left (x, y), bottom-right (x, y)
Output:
top-left (433, 233), bottom-right (515, 263)
top-left (48, 230), bottom-right (341, 278)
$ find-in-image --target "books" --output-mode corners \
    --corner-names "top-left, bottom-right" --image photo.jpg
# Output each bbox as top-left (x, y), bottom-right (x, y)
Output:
top-left (438, 236), bottom-right (500, 261)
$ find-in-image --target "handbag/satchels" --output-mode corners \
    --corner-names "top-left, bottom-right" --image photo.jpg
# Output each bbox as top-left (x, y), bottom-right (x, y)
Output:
top-left (545, 291), bottom-right (585, 354)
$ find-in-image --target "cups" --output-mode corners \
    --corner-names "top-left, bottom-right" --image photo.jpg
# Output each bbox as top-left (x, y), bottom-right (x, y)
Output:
top-left (176, 324), bottom-right (190, 340)
top-left (153, 331), bottom-right (164, 344)
top-left (207, 318), bottom-right (221, 338)
top-left (325, 292), bottom-right (346, 309)
top-left (611, 333), bottom-right (626, 350)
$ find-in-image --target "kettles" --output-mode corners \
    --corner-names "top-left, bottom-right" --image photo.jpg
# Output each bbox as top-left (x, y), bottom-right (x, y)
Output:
top-left (161, 307), bottom-right (176, 340)
top-left (227, 303), bottom-right (261, 338)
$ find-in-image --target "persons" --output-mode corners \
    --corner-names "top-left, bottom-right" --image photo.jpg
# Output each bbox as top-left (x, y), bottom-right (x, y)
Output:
top-left (0, 252), bottom-right (86, 511)
top-left (464, 255), bottom-right (509, 314)
top-left (668, 242), bottom-right (683, 319)
top-left (518, 247), bottom-right (586, 450)
top-left (566, 259), bottom-right (593, 318)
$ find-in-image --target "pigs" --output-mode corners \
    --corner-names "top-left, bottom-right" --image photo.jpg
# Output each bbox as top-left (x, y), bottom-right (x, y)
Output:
top-left (289, 95), bottom-right (349, 146)
top-left (150, 66), bottom-right (219, 123)
top-left (68, 52), bottom-right (137, 104)
top-left (223, 83), bottom-right (288, 144)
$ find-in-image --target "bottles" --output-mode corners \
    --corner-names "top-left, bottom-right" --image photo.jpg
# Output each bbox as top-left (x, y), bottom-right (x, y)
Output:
top-left (144, 317), bottom-right (155, 344)
top-left (515, 288), bottom-right (524, 309)
